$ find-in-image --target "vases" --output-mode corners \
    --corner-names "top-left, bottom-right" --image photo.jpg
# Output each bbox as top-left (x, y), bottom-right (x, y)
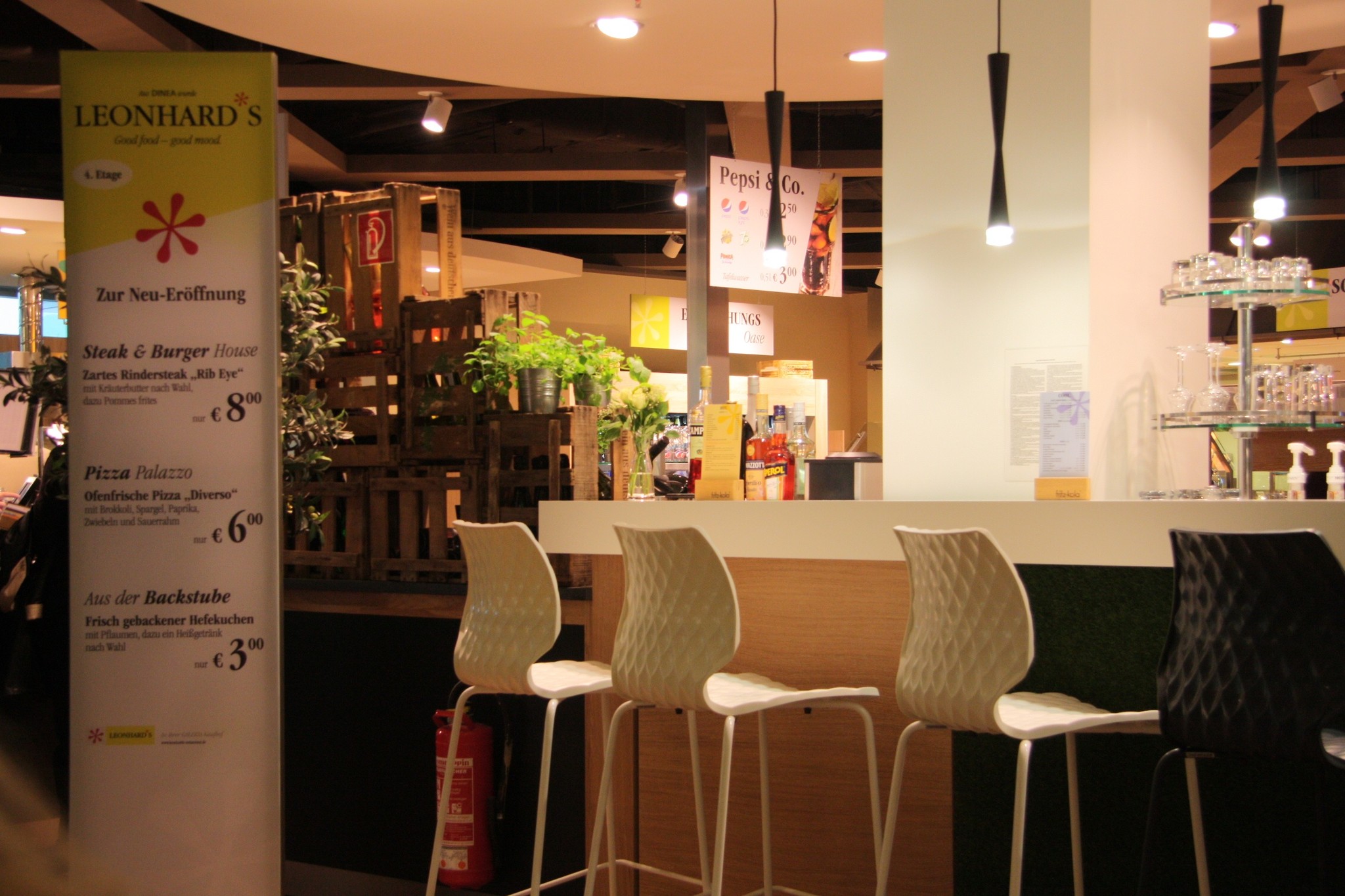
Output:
top-left (627, 436), bottom-right (656, 502)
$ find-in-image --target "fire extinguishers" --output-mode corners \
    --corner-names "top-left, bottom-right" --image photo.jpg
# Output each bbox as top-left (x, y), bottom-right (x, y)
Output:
top-left (427, 679), bottom-right (497, 893)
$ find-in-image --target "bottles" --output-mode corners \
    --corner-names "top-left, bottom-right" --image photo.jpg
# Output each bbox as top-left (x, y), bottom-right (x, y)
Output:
top-left (687, 366), bottom-right (818, 500)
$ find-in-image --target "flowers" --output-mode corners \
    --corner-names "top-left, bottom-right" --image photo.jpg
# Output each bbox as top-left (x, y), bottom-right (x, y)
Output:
top-left (620, 382), bottom-right (681, 499)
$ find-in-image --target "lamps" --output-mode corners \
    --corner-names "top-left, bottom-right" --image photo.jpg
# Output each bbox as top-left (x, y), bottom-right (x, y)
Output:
top-left (661, 231), bottom-right (684, 258)
top-left (418, 90), bottom-right (453, 133)
top-left (762, 0), bottom-right (789, 272)
top-left (673, 177), bottom-right (687, 207)
top-left (1252, 0), bottom-right (1287, 225)
top-left (1229, 224), bottom-right (1251, 247)
top-left (1252, 220), bottom-right (1271, 246)
top-left (1308, 74), bottom-right (1343, 113)
top-left (984, 0), bottom-right (1016, 248)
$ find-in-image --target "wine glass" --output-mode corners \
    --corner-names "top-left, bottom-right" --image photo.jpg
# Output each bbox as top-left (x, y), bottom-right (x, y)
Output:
top-left (1188, 342), bottom-right (1231, 424)
top-left (1161, 344), bottom-right (1200, 414)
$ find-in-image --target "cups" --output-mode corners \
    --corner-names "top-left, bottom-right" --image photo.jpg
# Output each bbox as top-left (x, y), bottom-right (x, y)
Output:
top-left (1252, 362), bottom-right (1336, 423)
top-left (1170, 250), bottom-right (1317, 293)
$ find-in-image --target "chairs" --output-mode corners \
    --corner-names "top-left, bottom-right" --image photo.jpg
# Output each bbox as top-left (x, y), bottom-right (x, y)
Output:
top-left (874, 526), bottom-right (1159, 894)
top-left (584, 523), bottom-right (883, 896)
top-left (427, 518), bottom-right (712, 896)
top-left (1159, 528), bottom-right (1344, 896)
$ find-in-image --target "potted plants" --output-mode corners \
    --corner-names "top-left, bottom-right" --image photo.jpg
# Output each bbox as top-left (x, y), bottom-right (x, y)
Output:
top-left (461, 310), bottom-right (651, 415)
